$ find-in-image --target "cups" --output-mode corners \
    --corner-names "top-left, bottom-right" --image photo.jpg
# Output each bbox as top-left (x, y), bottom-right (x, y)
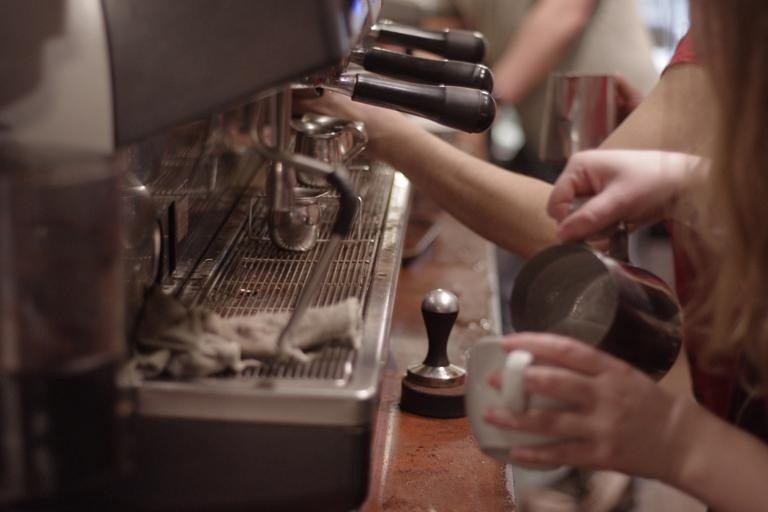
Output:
top-left (538, 72), bottom-right (618, 180)
top-left (506, 197), bottom-right (683, 387)
top-left (466, 339), bottom-right (565, 472)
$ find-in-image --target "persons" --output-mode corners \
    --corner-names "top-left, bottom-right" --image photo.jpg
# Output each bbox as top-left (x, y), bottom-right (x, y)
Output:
top-left (415, 0), bottom-right (662, 183)
top-left (314, 33), bottom-right (719, 261)
top-left (479, 0), bottom-right (768, 511)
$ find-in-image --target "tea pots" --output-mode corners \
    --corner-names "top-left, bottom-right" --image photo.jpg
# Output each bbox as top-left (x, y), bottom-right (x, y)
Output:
top-left (288, 119), bottom-right (368, 188)
